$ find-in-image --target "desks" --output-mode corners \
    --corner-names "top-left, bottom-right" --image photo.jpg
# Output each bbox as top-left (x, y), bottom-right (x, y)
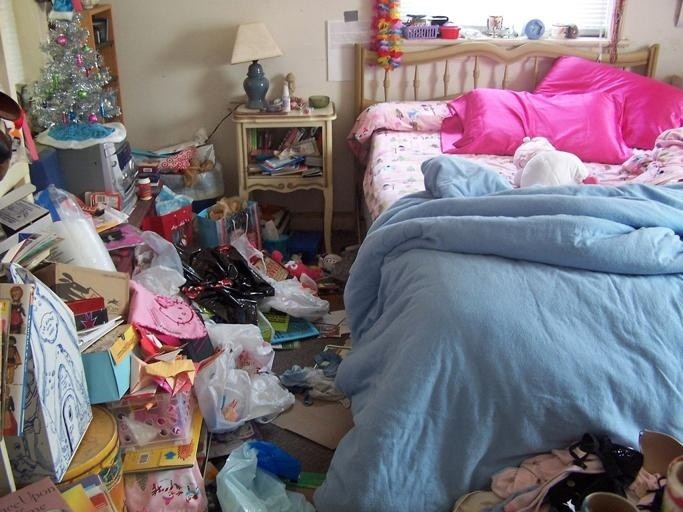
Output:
top-left (124, 186), bottom-right (162, 233)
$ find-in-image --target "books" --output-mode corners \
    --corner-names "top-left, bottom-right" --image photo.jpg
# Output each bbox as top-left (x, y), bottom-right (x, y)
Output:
top-left (246, 127), bottom-right (322, 177)
top-left (256, 310), bottom-right (320, 351)
top-left (0, 282), bottom-right (35, 436)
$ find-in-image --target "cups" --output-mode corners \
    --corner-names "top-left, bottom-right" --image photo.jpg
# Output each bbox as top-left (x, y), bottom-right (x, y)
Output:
top-left (488, 16), bottom-right (502, 36)
top-left (551, 26), bottom-right (569, 38)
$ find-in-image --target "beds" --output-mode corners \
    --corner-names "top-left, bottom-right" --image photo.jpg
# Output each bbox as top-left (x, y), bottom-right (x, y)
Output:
top-left (309, 39), bottom-right (683, 511)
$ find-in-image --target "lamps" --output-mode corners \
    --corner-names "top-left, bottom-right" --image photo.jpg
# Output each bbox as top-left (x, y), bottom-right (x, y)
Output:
top-left (229, 20), bottom-right (282, 109)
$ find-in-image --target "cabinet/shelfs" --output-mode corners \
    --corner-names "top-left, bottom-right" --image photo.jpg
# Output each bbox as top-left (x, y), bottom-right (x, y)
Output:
top-left (232, 98), bottom-right (337, 255)
top-left (79, 4), bottom-right (121, 124)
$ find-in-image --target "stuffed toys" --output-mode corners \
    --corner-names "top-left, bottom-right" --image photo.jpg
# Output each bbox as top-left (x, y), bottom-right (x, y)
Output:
top-left (513, 137), bottom-right (598, 188)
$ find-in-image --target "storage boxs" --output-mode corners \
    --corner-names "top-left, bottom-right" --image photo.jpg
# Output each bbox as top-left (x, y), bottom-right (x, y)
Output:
top-left (82, 324), bottom-right (135, 406)
top-left (31, 259), bottom-right (131, 321)
top-left (0, 198), bottom-right (54, 257)
top-left (0, 262), bottom-right (93, 485)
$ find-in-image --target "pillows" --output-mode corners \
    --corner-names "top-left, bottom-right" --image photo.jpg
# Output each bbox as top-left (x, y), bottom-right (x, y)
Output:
top-left (439, 87), bottom-right (632, 164)
top-left (532, 53), bottom-right (683, 152)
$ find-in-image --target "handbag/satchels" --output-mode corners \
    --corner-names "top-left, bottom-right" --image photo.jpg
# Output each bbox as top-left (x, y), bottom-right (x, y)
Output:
top-left (179, 245), bottom-right (274, 326)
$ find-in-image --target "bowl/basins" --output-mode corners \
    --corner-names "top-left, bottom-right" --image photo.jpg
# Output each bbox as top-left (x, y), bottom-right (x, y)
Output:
top-left (309, 96), bottom-right (330, 108)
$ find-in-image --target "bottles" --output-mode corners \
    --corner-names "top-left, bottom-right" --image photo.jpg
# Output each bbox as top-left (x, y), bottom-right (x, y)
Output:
top-left (137, 173), bottom-right (152, 201)
